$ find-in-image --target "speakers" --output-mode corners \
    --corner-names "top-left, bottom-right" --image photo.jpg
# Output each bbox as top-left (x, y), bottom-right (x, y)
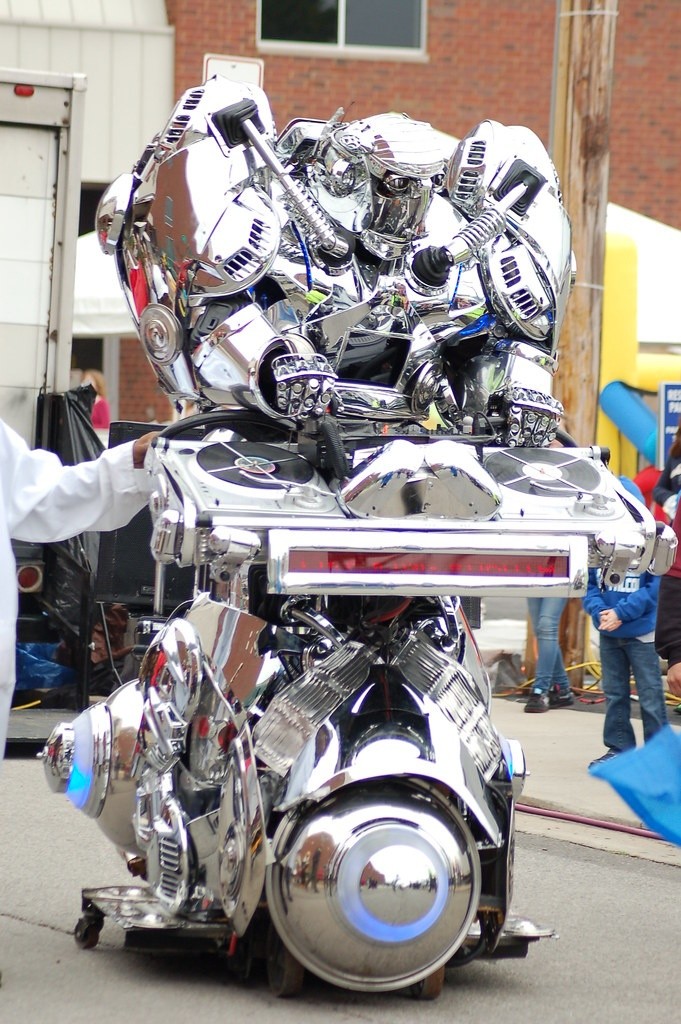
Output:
top-left (94, 421), bottom-right (205, 608)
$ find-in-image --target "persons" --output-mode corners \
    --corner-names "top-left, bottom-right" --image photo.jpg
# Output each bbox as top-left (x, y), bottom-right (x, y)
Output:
top-left (79, 370), bottom-right (111, 430)
top-left (0, 417), bottom-right (162, 774)
top-left (524, 411), bottom-right (681, 769)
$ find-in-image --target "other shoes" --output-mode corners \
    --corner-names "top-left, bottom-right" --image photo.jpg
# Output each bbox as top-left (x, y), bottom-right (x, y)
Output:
top-left (547, 684), bottom-right (574, 709)
top-left (588, 749), bottom-right (621, 769)
top-left (524, 687), bottom-right (549, 712)
